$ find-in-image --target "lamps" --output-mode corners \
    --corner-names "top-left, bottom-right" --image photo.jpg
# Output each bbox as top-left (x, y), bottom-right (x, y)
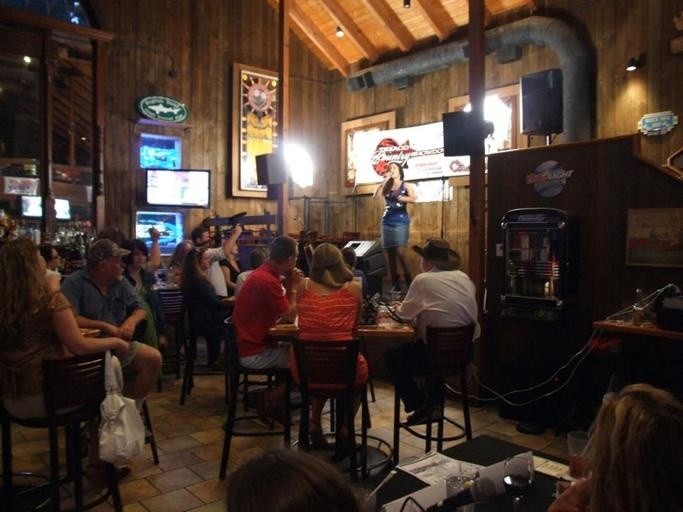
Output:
top-left (621, 51), bottom-right (645, 74)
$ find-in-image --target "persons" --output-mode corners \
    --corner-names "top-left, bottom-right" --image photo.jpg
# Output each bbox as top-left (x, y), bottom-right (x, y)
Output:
top-left (223, 449), bottom-right (371, 511)
top-left (0, 232), bottom-right (136, 479)
top-left (171, 220), bottom-right (242, 369)
top-left (39, 227), bottom-right (161, 437)
top-left (544, 381), bottom-right (682, 511)
top-left (372, 161), bottom-right (417, 295)
top-left (383, 235), bottom-right (481, 425)
top-left (234, 235), bottom-right (370, 450)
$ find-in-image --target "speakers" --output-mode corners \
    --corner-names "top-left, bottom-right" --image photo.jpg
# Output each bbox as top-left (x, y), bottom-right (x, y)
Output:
top-left (442, 111), bottom-right (483, 157)
top-left (393, 75), bottom-right (413, 90)
top-left (255, 153), bottom-right (286, 185)
top-left (494, 44), bottom-right (522, 63)
top-left (520, 68), bottom-right (563, 136)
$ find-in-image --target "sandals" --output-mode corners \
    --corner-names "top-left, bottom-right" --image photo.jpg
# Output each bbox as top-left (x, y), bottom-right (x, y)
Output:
top-left (308, 417), bottom-right (330, 448)
top-left (336, 423), bottom-right (353, 455)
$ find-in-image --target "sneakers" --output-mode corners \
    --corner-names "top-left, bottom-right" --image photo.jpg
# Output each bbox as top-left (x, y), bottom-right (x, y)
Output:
top-left (277, 406), bottom-right (294, 428)
top-left (406, 403), bottom-right (443, 426)
top-left (256, 390), bottom-right (276, 431)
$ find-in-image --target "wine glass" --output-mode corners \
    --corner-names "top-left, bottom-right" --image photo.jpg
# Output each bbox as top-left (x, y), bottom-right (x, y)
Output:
top-left (502, 456), bottom-right (532, 509)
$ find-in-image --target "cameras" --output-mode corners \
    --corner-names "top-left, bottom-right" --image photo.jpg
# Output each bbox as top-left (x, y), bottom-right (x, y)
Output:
top-left (224, 222), bottom-right (245, 236)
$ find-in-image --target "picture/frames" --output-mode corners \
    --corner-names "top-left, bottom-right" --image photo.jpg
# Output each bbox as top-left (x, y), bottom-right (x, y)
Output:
top-left (335, 106), bottom-right (400, 200)
top-left (440, 77), bottom-right (531, 190)
top-left (225, 58), bottom-right (284, 202)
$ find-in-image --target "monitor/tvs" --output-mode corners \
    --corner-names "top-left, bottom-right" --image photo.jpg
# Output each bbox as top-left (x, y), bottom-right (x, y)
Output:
top-left (54, 197), bottom-right (71, 220)
top-left (137, 131), bottom-right (184, 171)
top-left (17, 194), bottom-right (44, 219)
top-left (131, 206), bottom-right (186, 256)
top-left (145, 167), bottom-right (211, 210)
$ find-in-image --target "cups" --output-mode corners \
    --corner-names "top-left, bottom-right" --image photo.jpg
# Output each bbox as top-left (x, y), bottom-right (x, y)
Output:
top-left (554, 474), bottom-right (574, 498)
top-left (444, 471), bottom-right (476, 499)
top-left (564, 431), bottom-right (592, 480)
top-left (459, 460), bottom-right (479, 481)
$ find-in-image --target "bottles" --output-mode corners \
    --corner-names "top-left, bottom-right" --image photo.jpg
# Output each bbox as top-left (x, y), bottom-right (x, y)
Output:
top-left (0, 208), bottom-right (96, 261)
top-left (632, 288), bottom-right (643, 326)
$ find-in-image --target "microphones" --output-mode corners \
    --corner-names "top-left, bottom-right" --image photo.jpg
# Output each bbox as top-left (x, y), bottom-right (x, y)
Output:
top-left (383, 171), bottom-right (392, 179)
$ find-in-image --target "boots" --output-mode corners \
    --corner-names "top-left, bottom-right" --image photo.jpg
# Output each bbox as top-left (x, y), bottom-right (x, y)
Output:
top-left (404, 273), bottom-right (413, 289)
top-left (388, 272), bottom-right (402, 294)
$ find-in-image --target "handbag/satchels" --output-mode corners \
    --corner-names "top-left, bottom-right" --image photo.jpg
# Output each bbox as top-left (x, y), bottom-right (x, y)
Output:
top-left (98, 348), bottom-right (146, 466)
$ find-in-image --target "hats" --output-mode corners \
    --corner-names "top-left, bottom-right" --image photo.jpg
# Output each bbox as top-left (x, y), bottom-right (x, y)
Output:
top-left (411, 236), bottom-right (462, 270)
top-left (86, 239), bottom-right (132, 264)
